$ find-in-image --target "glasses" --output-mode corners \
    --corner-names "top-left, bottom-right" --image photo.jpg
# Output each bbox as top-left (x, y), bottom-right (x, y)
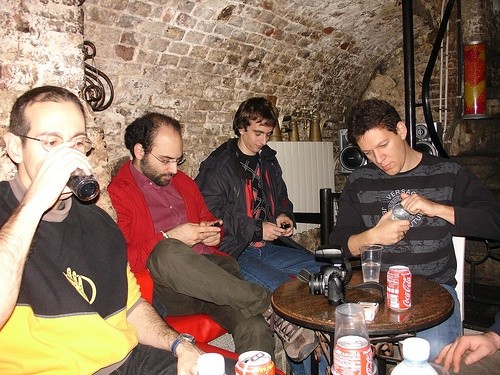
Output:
top-left (147, 148), bottom-right (186, 165)
top-left (17, 133), bottom-right (93, 153)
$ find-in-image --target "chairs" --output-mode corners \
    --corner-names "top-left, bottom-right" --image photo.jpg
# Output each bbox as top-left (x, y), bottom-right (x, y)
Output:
top-left (318, 188), bottom-right (465, 274)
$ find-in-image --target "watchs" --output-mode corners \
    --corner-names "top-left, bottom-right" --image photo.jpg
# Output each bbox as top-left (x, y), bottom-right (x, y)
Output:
top-left (171, 334), bottom-right (195, 357)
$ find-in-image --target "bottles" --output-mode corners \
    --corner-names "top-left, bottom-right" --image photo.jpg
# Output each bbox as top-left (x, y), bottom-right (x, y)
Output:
top-left (390, 337), bottom-right (439, 375)
top-left (391, 204), bottom-right (410, 221)
top-left (196, 352), bottom-right (228, 375)
top-left (267, 96), bottom-right (322, 142)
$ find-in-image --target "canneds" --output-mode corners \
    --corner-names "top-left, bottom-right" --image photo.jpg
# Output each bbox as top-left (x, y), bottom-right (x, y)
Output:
top-left (332, 334), bottom-right (373, 375)
top-left (235, 350), bottom-right (276, 375)
top-left (387, 265), bottom-right (412, 311)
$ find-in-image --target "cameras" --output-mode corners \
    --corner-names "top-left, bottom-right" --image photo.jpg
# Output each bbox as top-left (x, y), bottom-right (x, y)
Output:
top-left (307, 245), bottom-right (350, 305)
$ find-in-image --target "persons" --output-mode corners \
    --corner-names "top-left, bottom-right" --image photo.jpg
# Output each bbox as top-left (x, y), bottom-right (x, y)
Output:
top-left (195, 98), bottom-right (332, 375)
top-left (0, 85), bottom-right (238, 375)
top-left (334, 100), bottom-right (500, 375)
top-left (106, 112), bottom-right (318, 375)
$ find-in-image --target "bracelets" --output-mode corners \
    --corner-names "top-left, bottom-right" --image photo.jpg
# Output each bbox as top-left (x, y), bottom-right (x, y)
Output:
top-left (162, 231), bottom-right (168, 239)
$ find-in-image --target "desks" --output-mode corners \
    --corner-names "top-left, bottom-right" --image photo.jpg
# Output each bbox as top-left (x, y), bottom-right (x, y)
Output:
top-left (271, 272), bottom-right (454, 375)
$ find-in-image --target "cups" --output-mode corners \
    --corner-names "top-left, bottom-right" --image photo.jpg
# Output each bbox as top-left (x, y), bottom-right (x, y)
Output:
top-left (359, 245), bottom-right (383, 285)
top-left (329, 302), bottom-right (378, 375)
top-left (66, 168), bottom-right (99, 201)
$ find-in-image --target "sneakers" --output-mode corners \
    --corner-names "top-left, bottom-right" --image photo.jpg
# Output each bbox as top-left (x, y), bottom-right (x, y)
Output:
top-left (261, 305), bottom-right (318, 364)
